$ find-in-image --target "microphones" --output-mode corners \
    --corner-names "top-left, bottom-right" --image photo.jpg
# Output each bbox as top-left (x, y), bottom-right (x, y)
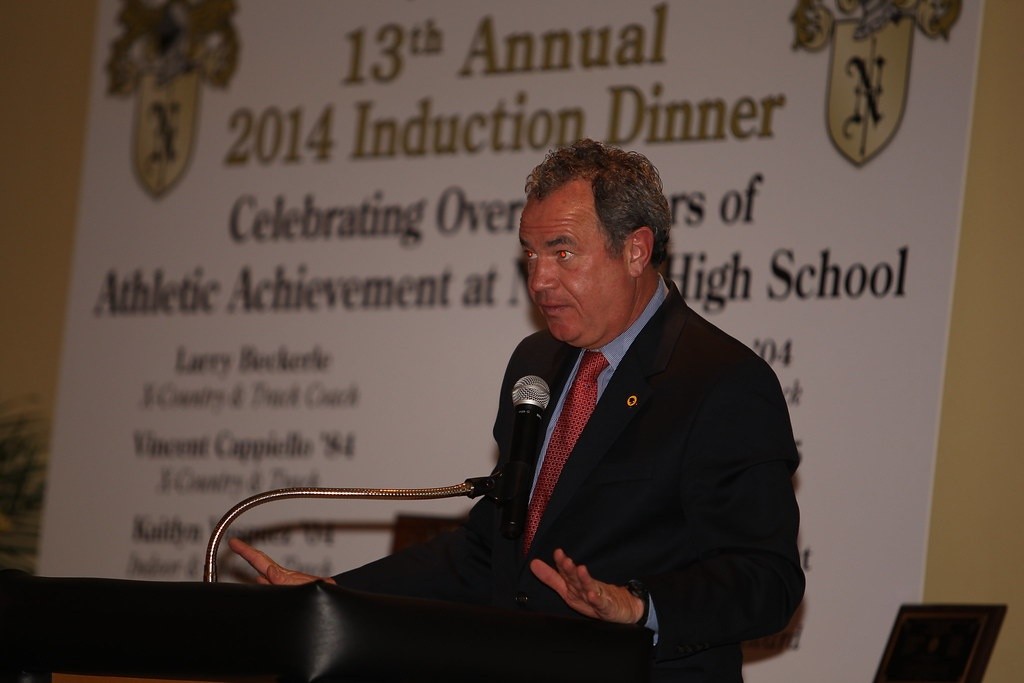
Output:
top-left (499, 376), bottom-right (550, 543)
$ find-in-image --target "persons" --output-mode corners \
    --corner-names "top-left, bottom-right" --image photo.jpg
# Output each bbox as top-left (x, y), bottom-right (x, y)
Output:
top-left (227, 137), bottom-right (805, 683)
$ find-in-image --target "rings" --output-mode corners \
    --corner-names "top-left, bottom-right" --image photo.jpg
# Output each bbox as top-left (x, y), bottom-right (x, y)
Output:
top-left (598, 587), bottom-right (603, 596)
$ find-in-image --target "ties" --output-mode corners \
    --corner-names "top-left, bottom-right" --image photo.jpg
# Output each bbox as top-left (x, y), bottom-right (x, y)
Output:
top-left (523, 351), bottom-right (609, 558)
